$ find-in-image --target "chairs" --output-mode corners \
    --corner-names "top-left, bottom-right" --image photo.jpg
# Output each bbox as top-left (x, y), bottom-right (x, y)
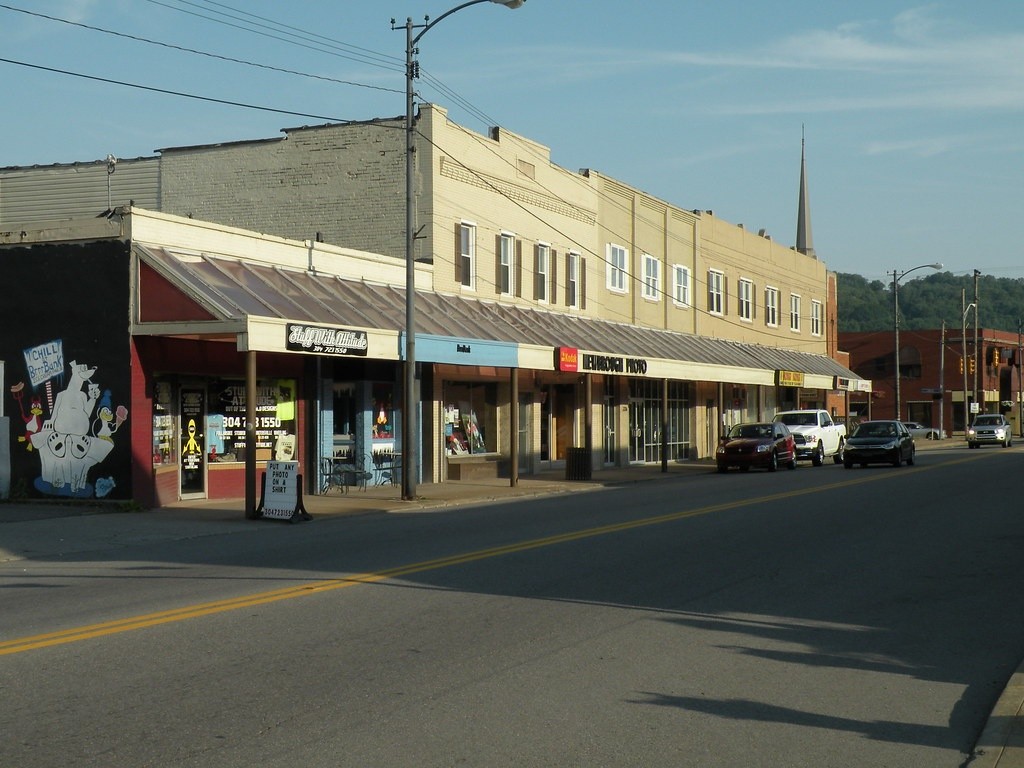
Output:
top-left (372, 453), bottom-right (403, 489)
top-left (318, 452), bottom-right (371, 495)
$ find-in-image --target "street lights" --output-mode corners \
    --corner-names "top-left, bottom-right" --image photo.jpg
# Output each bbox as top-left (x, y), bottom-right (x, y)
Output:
top-left (893, 261), bottom-right (944, 421)
top-left (402, 0), bottom-right (525, 503)
top-left (961, 288), bottom-right (977, 438)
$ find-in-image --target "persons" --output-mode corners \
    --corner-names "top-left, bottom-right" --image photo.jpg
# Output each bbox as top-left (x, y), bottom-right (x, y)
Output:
top-left (883, 424), bottom-right (897, 436)
top-left (992, 417), bottom-right (1001, 424)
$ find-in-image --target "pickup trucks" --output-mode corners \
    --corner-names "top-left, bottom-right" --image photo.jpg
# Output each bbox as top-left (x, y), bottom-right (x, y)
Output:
top-left (770, 409), bottom-right (846, 467)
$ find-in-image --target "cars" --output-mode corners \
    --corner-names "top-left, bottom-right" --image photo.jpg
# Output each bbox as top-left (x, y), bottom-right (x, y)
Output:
top-left (966, 414), bottom-right (1012, 449)
top-left (716, 421), bottom-right (796, 473)
top-left (843, 420), bottom-right (916, 467)
top-left (901, 422), bottom-right (947, 440)
